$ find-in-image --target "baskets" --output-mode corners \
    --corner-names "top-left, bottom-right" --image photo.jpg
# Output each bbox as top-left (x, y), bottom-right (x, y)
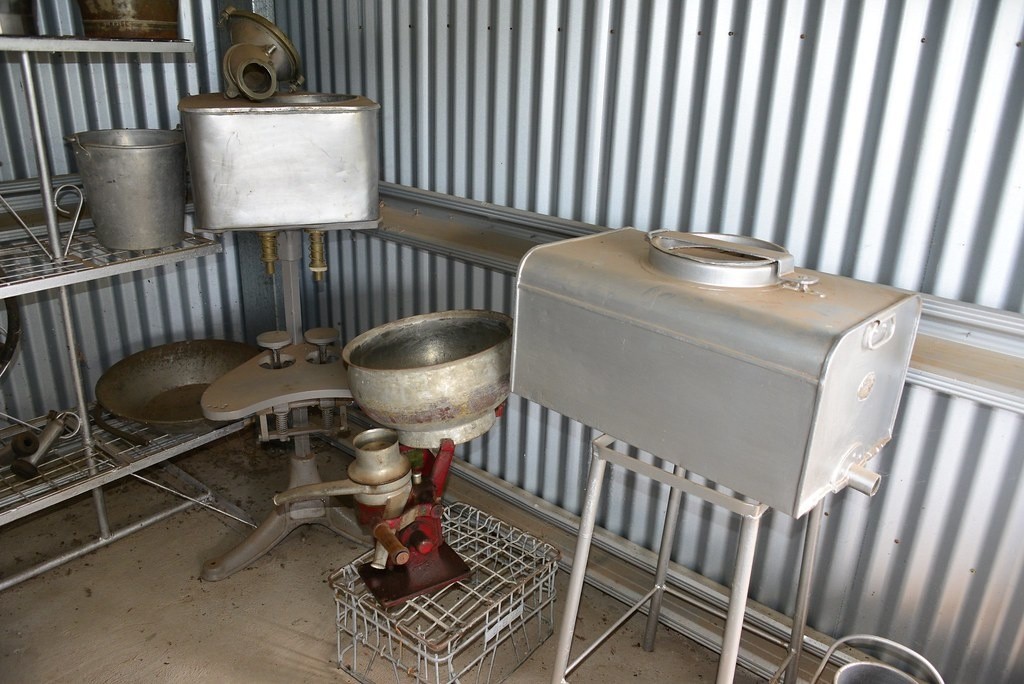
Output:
top-left (328, 501), bottom-right (561, 684)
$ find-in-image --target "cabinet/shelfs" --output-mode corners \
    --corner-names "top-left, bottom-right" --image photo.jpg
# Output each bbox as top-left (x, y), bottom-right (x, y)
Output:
top-left (0, 34), bottom-right (258, 591)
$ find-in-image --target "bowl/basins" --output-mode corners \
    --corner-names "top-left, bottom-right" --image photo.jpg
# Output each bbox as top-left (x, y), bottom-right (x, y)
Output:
top-left (94, 339), bottom-right (266, 433)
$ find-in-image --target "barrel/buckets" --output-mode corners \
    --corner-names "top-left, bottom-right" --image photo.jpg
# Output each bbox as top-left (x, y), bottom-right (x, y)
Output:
top-left (64, 122), bottom-right (190, 252)
